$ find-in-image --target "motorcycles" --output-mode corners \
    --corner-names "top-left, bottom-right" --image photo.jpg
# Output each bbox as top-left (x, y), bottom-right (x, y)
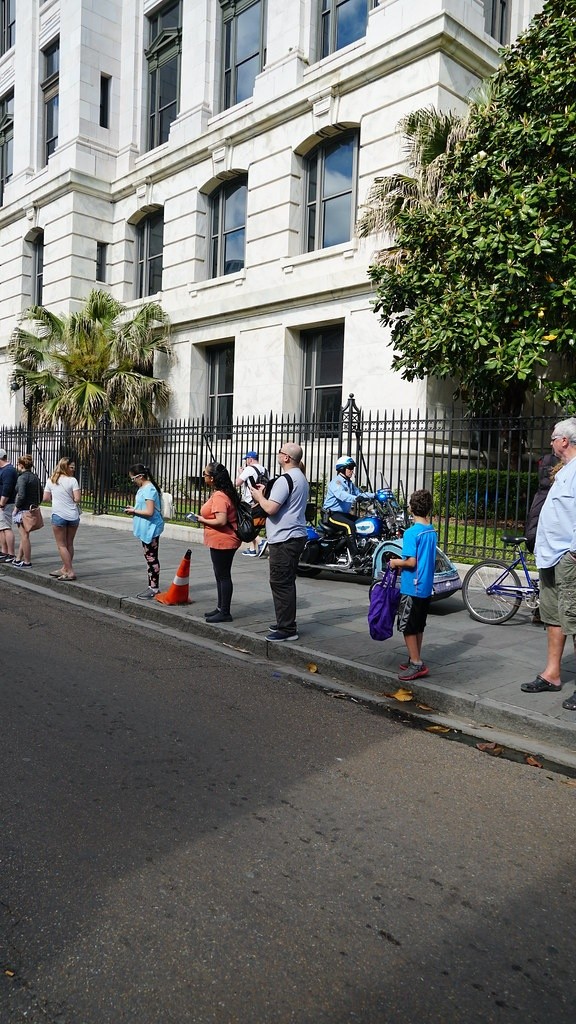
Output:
top-left (297, 487), bottom-right (412, 584)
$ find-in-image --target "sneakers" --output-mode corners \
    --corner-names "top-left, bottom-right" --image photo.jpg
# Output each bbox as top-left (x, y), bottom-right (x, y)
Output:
top-left (258, 540), bottom-right (268, 557)
top-left (400, 656), bottom-right (411, 669)
top-left (398, 660), bottom-right (428, 680)
top-left (265, 631), bottom-right (298, 642)
top-left (205, 609), bottom-right (220, 617)
top-left (0, 551), bottom-right (76, 580)
top-left (206, 612), bottom-right (233, 622)
top-left (242, 549), bottom-right (256, 557)
top-left (269, 625), bottom-right (277, 632)
top-left (136, 588), bottom-right (161, 600)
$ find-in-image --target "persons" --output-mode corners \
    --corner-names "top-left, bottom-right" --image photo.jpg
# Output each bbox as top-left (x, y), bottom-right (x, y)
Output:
top-left (0, 449), bottom-right (18, 563)
top-left (525, 454), bottom-right (564, 624)
top-left (12, 455), bottom-right (43, 568)
top-left (236, 451), bottom-right (268, 557)
top-left (387, 490), bottom-right (437, 680)
top-left (194, 462), bottom-right (243, 623)
top-left (43, 457), bottom-right (81, 580)
top-left (124, 464), bottom-right (164, 599)
top-left (321, 456), bottom-right (375, 568)
top-left (250, 443), bottom-right (309, 642)
top-left (521, 418), bottom-right (576, 709)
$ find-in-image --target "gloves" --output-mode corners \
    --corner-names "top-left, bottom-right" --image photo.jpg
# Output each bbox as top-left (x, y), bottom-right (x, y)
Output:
top-left (356, 496), bottom-right (363, 502)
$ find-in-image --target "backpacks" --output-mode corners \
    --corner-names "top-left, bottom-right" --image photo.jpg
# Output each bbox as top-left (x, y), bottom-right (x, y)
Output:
top-left (260, 473), bottom-right (294, 517)
top-left (154, 489), bottom-right (175, 521)
top-left (226, 493), bottom-right (257, 544)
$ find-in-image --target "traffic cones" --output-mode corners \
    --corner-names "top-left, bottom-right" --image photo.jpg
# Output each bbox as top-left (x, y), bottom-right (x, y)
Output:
top-left (156, 548), bottom-right (194, 605)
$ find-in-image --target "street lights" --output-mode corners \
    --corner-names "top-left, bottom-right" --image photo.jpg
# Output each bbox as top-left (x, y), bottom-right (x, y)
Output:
top-left (12, 373), bottom-right (33, 455)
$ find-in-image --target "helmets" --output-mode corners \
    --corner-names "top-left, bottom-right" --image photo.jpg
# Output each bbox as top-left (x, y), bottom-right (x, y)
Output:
top-left (336, 456), bottom-right (356, 470)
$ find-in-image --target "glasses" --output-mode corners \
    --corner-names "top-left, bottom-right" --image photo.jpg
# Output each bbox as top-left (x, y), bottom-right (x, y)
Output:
top-left (551, 436), bottom-right (562, 441)
top-left (203, 471), bottom-right (211, 476)
top-left (345, 465), bottom-right (354, 470)
top-left (69, 466), bottom-right (75, 469)
top-left (131, 474), bottom-right (144, 481)
top-left (279, 449), bottom-right (292, 458)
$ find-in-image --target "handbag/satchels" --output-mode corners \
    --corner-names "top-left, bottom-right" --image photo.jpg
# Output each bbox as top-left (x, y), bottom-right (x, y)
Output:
top-left (367, 564), bottom-right (401, 641)
top-left (77, 503), bottom-right (82, 515)
top-left (21, 504), bottom-right (44, 532)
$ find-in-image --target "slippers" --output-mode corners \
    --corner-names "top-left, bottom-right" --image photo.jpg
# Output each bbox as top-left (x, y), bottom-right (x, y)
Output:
top-left (562, 690), bottom-right (576, 709)
top-left (521, 675), bottom-right (561, 692)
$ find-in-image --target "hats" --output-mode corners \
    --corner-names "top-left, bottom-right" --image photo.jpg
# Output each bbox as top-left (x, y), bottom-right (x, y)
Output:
top-left (242, 452), bottom-right (258, 460)
top-left (0, 449), bottom-right (7, 459)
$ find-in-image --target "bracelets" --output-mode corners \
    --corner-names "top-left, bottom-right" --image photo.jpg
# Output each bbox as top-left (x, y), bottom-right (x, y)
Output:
top-left (134, 510), bottom-right (135, 514)
top-left (0, 505), bottom-right (5, 508)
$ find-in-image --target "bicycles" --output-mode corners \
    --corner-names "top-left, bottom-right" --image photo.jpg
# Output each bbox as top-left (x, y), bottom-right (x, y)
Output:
top-left (461, 536), bottom-right (546, 631)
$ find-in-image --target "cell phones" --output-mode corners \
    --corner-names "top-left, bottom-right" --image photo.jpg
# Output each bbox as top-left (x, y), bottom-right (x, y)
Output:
top-left (120, 507), bottom-right (126, 510)
top-left (248, 476), bottom-right (259, 491)
top-left (187, 512), bottom-right (197, 522)
